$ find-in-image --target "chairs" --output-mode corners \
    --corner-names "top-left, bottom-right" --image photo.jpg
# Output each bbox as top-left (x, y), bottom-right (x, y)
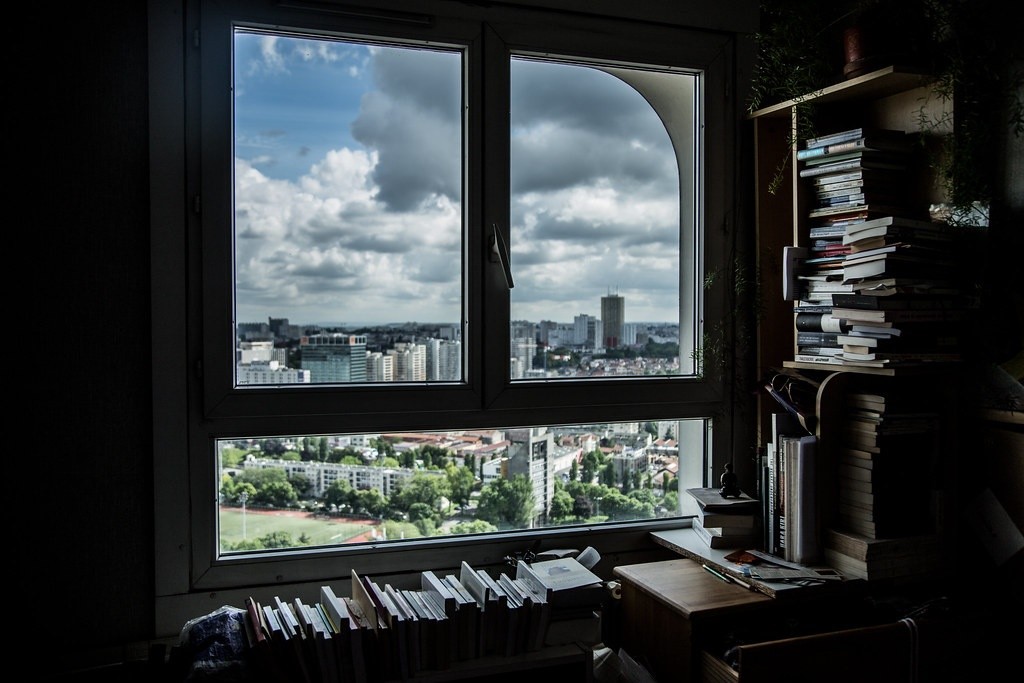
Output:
top-left (738, 618), bottom-right (918, 683)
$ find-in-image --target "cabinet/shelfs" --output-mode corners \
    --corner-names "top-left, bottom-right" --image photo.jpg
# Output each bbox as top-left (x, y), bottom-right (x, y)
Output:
top-left (746, 65), bottom-right (953, 570)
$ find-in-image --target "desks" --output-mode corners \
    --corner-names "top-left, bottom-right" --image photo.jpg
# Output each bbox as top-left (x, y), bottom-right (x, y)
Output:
top-left (408, 643), bottom-right (594, 683)
top-left (612, 557), bottom-right (963, 683)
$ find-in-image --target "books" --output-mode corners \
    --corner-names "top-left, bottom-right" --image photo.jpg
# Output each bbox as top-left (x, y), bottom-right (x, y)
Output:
top-left (240, 556), bottom-right (623, 679)
top-left (752, 124), bottom-right (976, 586)
